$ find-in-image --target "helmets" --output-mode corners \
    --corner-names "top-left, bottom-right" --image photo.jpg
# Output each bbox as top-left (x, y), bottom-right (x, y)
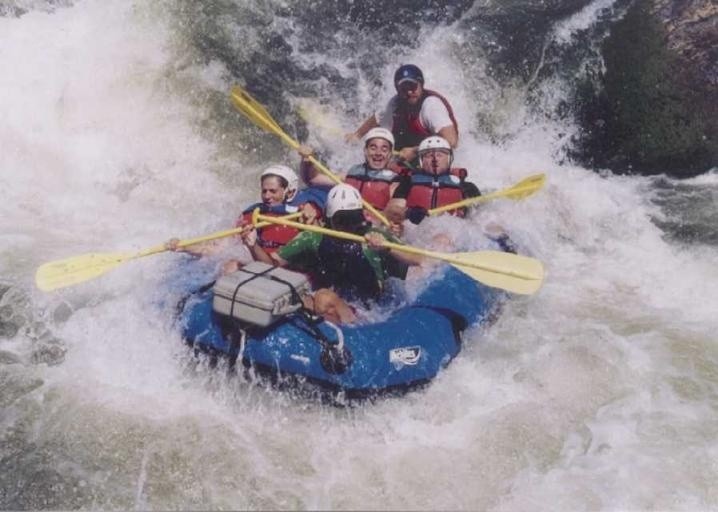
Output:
top-left (325, 183), bottom-right (363, 218)
top-left (417, 135), bottom-right (451, 168)
top-left (262, 165), bottom-right (299, 203)
top-left (361, 127), bottom-right (395, 165)
top-left (394, 65), bottom-right (422, 86)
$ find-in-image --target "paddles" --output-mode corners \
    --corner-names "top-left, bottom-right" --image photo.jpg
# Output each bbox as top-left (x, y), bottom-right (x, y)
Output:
top-left (252, 208), bottom-right (543, 295)
top-left (231, 86), bottom-right (388, 225)
top-left (427, 174), bottom-right (544, 217)
top-left (34, 212), bottom-right (304, 290)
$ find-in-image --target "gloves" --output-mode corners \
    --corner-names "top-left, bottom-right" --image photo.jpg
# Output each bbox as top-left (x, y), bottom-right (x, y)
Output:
top-left (405, 206), bottom-right (426, 224)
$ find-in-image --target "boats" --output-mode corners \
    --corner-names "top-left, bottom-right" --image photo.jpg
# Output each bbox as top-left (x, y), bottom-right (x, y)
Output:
top-left (172, 183), bottom-right (516, 410)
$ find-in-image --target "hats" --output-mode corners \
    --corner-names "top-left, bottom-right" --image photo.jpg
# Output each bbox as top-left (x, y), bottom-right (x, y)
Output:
top-left (395, 69), bottom-right (418, 85)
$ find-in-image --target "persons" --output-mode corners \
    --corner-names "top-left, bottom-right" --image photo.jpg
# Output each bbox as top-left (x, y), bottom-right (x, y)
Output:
top-left (384, 134), bottom-right (506, 251)
top-left (240, 183), bottom-right (422, 325)
top-left (298, 127), bottom-right (403, 239)
top-left (164, 165), bottom-right (327, 282)
top-left (344, 64), bottom-right (459, 162)
